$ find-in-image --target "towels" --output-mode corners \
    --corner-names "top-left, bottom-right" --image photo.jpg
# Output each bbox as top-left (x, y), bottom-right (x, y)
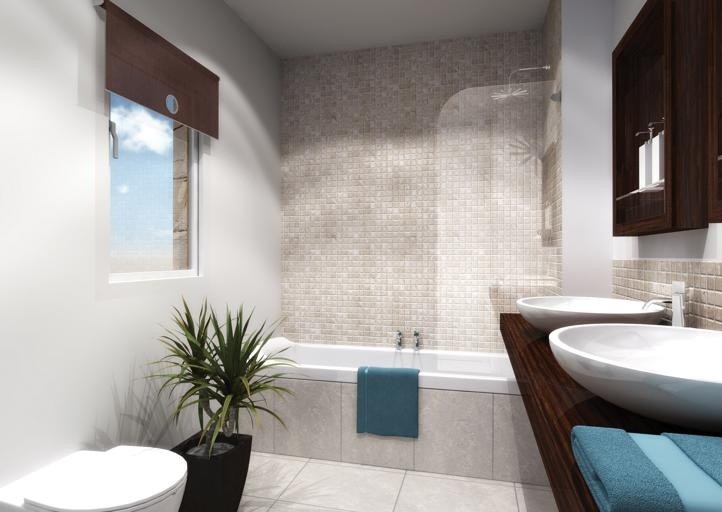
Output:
top-left (569, 420), bottom-right (721, 512)
top-left (356, 364), bottom-right (420, 438)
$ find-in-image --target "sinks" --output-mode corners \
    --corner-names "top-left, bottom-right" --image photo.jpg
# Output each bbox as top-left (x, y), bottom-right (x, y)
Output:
top-left (516, 296), bottom-right (666, 334)
top-left (548, 322), bottom-right (722, 429)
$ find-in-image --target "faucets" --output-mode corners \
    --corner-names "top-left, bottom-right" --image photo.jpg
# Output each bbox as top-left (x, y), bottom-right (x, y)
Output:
top-left (643, 282), bottom-right (687, 328)
top-left (412, 331), bottom-right (420, 350)
top-left (395, 331), bottom-right (403, 350)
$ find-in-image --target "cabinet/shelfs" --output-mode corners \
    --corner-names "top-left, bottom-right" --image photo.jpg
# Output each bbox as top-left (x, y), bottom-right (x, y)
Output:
top-left (609, 0), bottom-right (722, 237)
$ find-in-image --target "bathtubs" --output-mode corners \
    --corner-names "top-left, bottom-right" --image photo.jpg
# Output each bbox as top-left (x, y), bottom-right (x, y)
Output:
top-left (237, 337), bottom-right (551, 396)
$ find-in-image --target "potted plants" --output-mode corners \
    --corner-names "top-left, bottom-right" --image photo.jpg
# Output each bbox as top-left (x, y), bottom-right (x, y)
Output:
top-left (131, 292), bottom-right (299, 512)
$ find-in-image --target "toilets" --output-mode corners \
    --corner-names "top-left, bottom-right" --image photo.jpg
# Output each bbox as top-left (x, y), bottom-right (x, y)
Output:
top-left (0, 444), bottom-right (189, 512)
